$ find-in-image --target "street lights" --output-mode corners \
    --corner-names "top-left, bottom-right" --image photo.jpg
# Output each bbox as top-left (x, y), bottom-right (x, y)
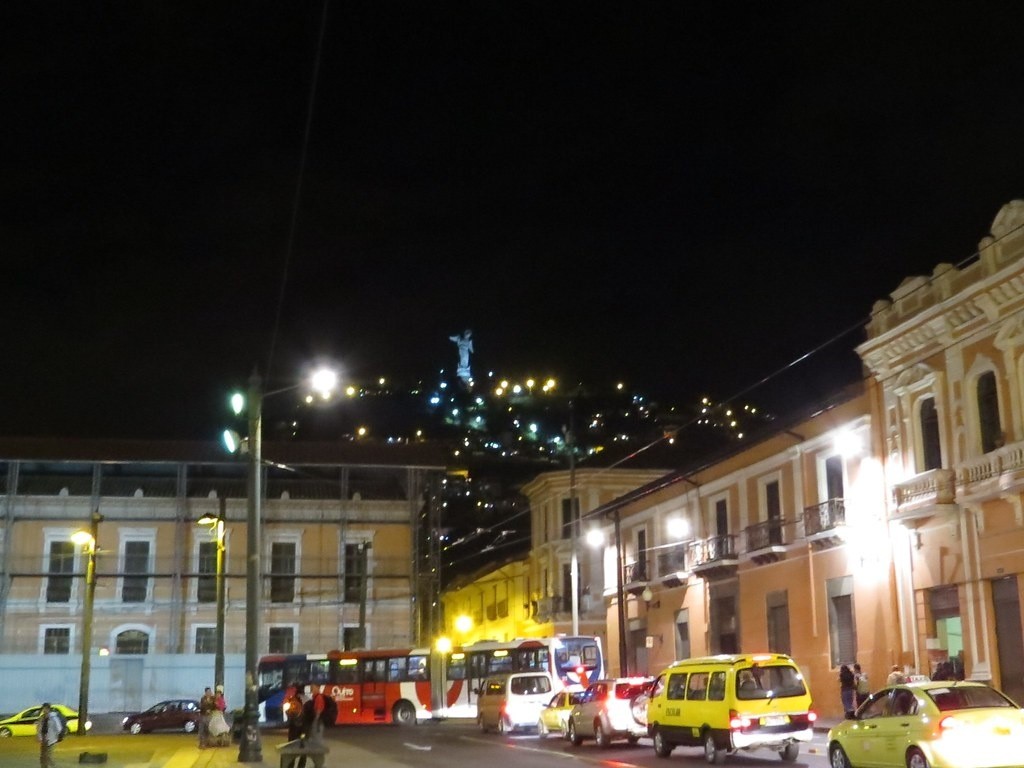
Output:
top-left (587, 511), bottom-right (629, 677)
top-left (196, 490), bottom-right (227, 690)
top-left (69, 510), bottom-right (106, 734)
top-left (236, 363), bottom-right (341, 763)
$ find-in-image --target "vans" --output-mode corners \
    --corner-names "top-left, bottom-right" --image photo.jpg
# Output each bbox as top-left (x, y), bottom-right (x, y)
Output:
top-left (473, 672), bottom-right (555, 737)
top-left (645, 652), bottom-right (816, 765)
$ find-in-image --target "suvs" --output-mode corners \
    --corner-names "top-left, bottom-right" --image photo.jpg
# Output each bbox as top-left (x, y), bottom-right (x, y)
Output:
top-left (567, 676), bottom-right (664, 748)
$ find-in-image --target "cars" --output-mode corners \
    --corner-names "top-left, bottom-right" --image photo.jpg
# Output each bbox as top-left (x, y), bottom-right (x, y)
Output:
top-left (121, 699), bottom-right (202, 734)
top-left (0, 705), bottom-right (94, 737)
top-left (538, 687), bottom-right (586, 740)
top-left (825, 679), bottom-right (1023, 768)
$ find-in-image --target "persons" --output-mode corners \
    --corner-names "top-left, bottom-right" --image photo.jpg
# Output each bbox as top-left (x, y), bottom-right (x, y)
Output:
top-left (933, 658), bottom-right (966, 682)
top-left (887, 665), bottom-right (906, 686)
top-left (449, 329), bottom-right (474, 368)
top-left (36, 703), bottom-right (63, 768)
top-left (286, 681), bottom-right (326, 768)
top-left (838, 663), bottom-right (871, 714)
top-left (198, 684), bottom-right (226, 749)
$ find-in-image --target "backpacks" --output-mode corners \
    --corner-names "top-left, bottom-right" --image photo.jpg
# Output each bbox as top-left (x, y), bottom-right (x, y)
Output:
top-left (858, 673), bottom-right (870, 695)
top-left (322, 694), bottom-right (338, 728)
top-left (894, 673), bottom-right (905, 685)
top-left (55, 711), bottom-right (66, 741)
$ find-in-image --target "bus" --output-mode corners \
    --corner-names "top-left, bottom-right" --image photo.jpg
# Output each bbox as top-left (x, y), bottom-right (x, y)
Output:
top-left (257, 634), bottom-right (605, 728)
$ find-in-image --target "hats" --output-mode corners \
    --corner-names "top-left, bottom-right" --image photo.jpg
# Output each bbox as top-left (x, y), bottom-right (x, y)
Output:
top-left (216, 685), bottom-right (224, 692)
